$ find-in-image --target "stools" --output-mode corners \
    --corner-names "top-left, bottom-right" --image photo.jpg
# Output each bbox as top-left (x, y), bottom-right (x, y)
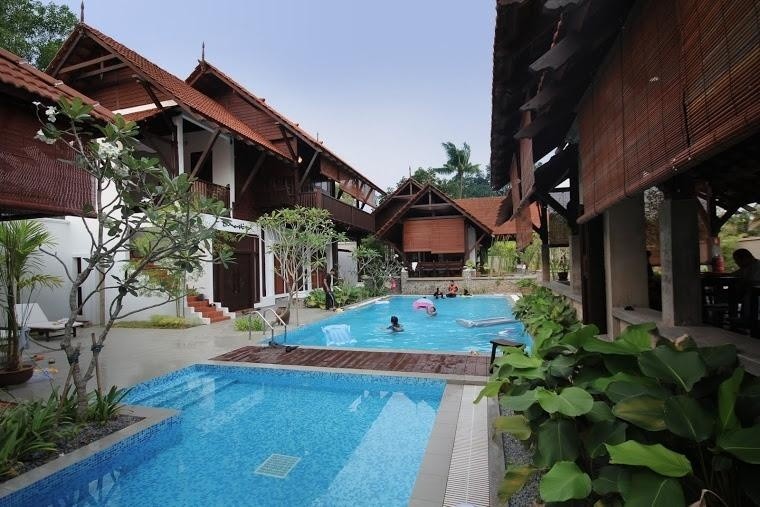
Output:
top-left (489, 338), bottom-right (524, 374)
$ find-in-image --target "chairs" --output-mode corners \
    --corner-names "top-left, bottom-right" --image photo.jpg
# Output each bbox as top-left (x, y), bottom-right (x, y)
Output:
top-left (701, 270), bottom-right (760, 336)
top-left (12, 302), bottom-right (84, 341)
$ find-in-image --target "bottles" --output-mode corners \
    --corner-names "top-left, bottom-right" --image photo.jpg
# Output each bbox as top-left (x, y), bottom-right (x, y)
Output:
top-left (710, 236), bottom-right (725, 273)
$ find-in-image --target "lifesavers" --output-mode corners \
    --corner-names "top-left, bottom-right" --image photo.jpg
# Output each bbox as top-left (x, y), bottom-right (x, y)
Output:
top-left (413, 299), bottom-right (437, 311)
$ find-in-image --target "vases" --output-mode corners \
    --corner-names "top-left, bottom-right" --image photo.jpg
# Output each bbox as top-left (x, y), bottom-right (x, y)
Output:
top-left (274, 307), bottom-right (290, 325)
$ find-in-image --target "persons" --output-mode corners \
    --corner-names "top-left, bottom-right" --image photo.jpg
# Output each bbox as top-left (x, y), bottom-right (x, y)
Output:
top-left (445, 279), bottom-right (458, 297)
top-left (434, 287), bottom-right (446, 299)
top-left (729, 247), bottom-right (760, 312)
top-left (386, 315), bottom-right (404, 331)
top-left (426, 305), bottom-right (437, 316)
top-left (322, 267), bottom-right (337, 310)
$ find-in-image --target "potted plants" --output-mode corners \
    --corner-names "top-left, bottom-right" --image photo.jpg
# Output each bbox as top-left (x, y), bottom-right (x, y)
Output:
top-left (557, 262), bottom-right (569, 281)
top-left (0, 218), bottom-right (65, 387)
